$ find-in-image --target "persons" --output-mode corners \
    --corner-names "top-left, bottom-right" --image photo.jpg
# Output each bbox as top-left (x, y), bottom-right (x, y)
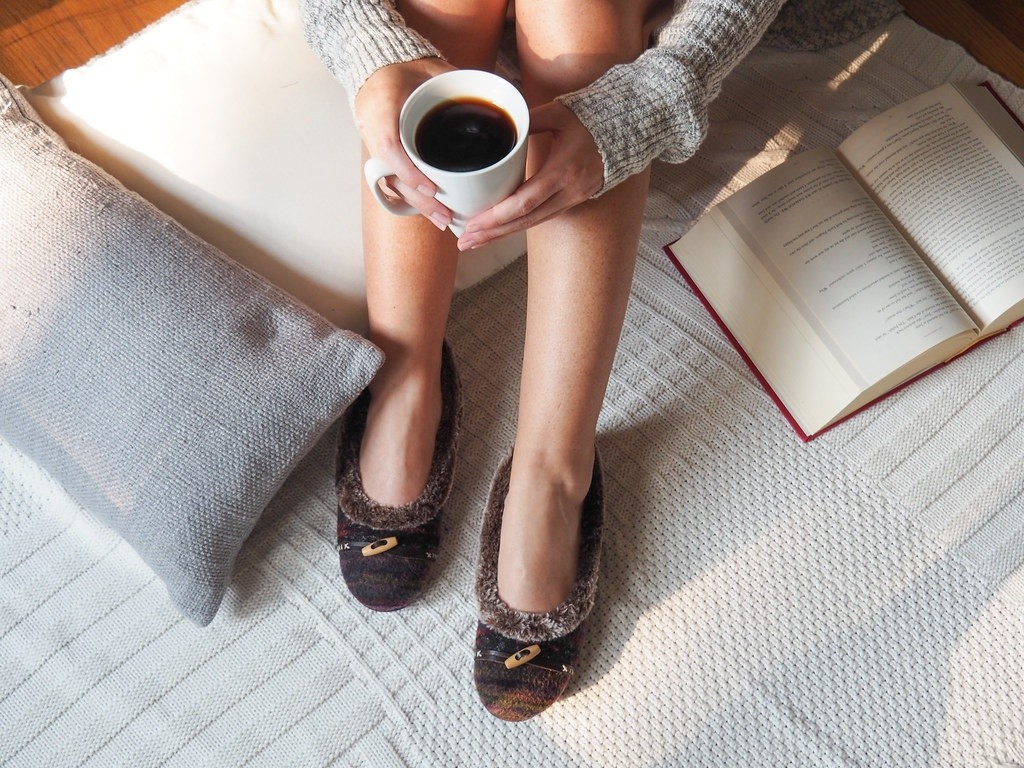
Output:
top-left (301, 1), bottom-right (784, 722)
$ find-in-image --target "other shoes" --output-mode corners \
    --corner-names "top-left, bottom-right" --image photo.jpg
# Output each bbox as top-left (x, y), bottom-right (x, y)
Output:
top-left (335, 324), bottom-right (463, 613)
top-left (473, 437), bottom-right (606, 722)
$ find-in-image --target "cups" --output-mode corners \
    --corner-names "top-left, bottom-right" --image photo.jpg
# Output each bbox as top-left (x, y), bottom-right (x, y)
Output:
top-left (365, 68), bottom-right (533, 239)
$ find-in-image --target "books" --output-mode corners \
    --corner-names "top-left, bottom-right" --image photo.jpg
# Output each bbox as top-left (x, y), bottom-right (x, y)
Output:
top-left (665, 79), bottom-right (1024, 441)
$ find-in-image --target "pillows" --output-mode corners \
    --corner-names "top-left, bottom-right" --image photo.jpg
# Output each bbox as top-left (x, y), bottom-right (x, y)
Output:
top-left (23, 1), bottom-right (534, 337)
top-left (0, 78), bottom-right (383, 626)
